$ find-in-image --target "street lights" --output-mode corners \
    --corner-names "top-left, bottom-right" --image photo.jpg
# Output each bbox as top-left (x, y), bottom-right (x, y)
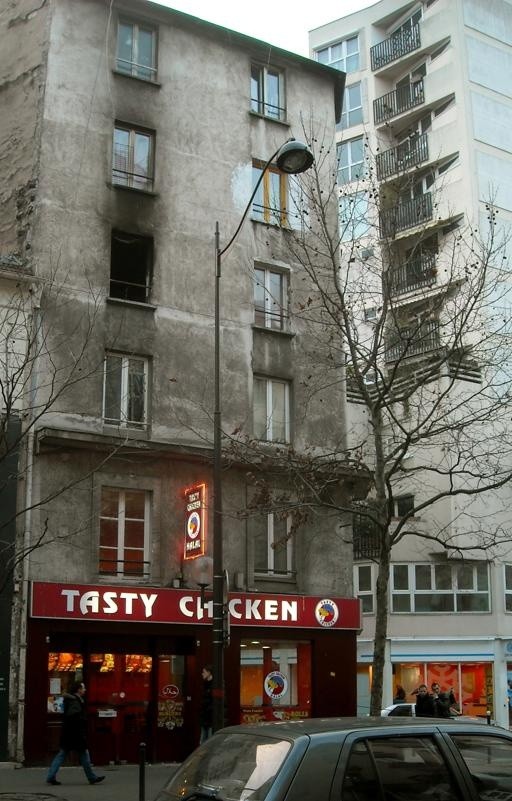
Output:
top-left (211, 136), bottom-right (316, 731)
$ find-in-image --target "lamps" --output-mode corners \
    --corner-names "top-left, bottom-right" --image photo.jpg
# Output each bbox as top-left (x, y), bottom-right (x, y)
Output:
top-left (328, 672), bottom-right (336, 680)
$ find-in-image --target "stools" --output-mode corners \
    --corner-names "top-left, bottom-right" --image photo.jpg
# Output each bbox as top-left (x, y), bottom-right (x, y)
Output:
top-left (96, 726), bottom-right (112, 759)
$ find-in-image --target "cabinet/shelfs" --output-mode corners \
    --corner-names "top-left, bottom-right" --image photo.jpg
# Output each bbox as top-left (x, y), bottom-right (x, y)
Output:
top-left (462, 668), bottom-right (493, 716)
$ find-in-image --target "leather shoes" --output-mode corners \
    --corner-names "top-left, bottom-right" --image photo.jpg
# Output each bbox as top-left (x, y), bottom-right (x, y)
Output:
top-left (87, 775), bottom-right (106, 784)
top-left (46, 776), bottom-right (61, 785)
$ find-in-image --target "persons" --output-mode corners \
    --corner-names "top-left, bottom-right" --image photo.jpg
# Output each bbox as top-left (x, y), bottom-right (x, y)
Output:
top-left (431, 682), bottom-right (451, 718)
top-left (45, 680), bottom-right (107, 785)
top-left (196, 663), bottom-right (217, 746)
top-left (396, 685), bottom-right (406, 700)
top-left (414, 685), bottom-right (433, 717)
top-left (448, 688), bottom-right (458, 708)
